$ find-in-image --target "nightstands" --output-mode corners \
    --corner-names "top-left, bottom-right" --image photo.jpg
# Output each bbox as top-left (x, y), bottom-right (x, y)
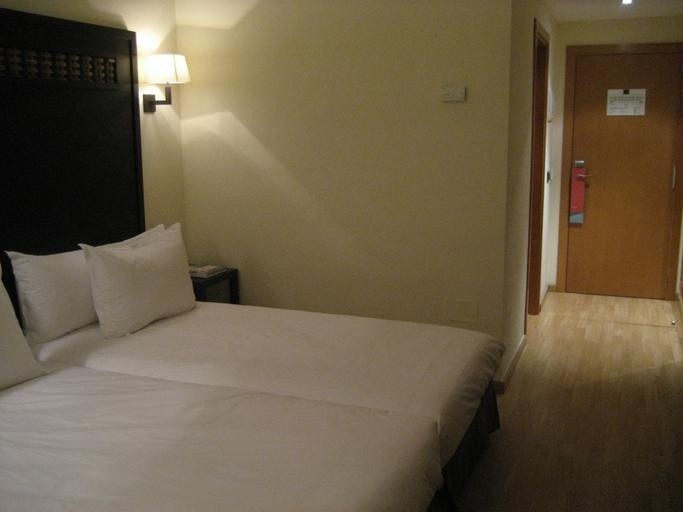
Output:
top-left (189, 267), bottom-right (239, 304)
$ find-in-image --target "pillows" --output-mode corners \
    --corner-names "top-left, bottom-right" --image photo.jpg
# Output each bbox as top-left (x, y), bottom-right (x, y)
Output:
top-left (0, 221), bottom-right (195, 391)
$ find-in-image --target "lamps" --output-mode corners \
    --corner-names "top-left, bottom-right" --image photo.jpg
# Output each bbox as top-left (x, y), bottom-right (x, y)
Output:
top-left (143, 54), bottom-right (190, 112)
